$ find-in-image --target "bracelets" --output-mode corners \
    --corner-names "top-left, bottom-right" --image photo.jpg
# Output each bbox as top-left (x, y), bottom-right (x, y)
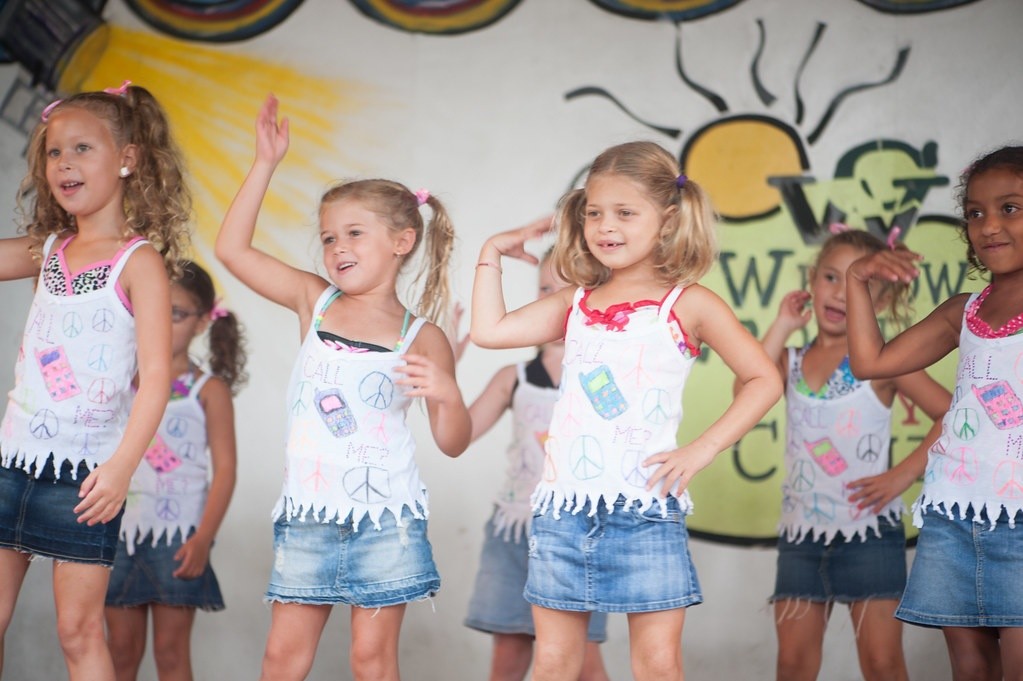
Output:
top-left (475, 262), bottom-right (503, 274)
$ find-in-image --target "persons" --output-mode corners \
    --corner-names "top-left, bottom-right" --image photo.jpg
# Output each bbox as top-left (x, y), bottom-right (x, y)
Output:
top-left (847, 146), bottom-right (1023, 681)
top-left (445, 142), bottom-right (784, 680)
top-left (0, 80), bottom-right (242, 681)
top-left (213, 92), bottom-right (471, 680)
top-left (730, 222), bottom-right (954, 681)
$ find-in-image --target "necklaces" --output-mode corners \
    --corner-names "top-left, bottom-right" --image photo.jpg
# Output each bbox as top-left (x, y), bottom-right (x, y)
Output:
top-left (966, 283), bottom-right (1023, 339)
